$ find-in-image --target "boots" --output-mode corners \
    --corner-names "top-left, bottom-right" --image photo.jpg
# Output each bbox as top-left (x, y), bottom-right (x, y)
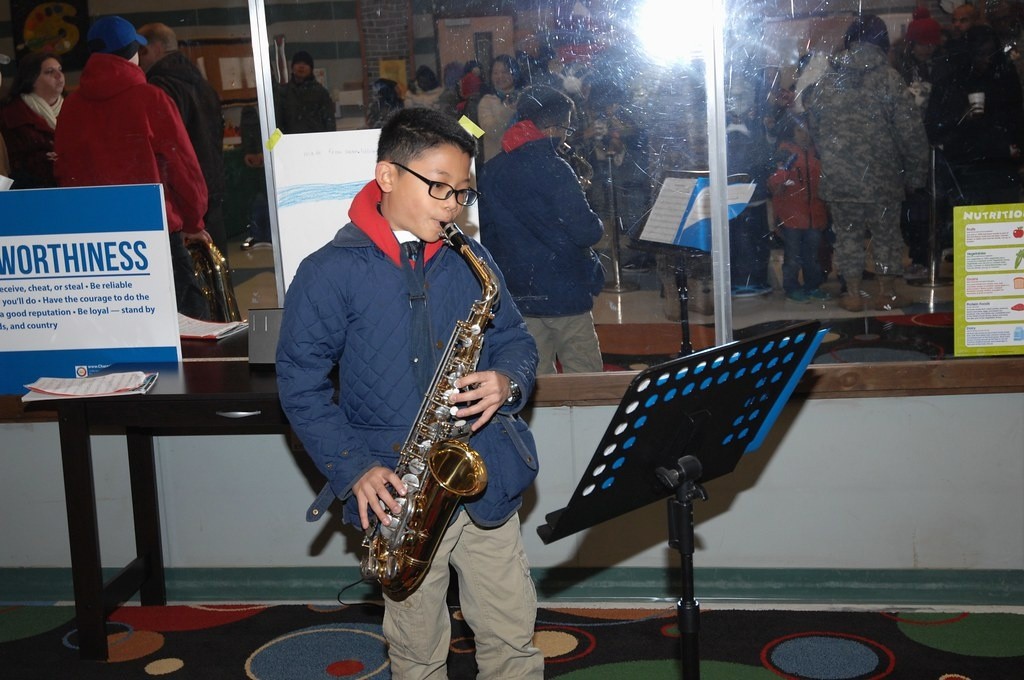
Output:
top-left (838, 277), bottom-right (863, 311)
top-left (687, 277), bottom-right (714, 316)
top-left (871, 275), bottom-right (912, 310)
top-left (657, 271), bottom-right (681, 321)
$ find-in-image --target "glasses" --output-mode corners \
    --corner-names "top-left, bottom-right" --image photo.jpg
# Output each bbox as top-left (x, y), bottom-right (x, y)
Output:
top-left (545, 124), bottom-right (575, 136)
top-left (389, 161), bottom-right (482, 206)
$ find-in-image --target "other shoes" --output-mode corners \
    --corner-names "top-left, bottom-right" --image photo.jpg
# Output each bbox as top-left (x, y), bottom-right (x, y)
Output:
top-left (621, 254), bottom-right (656, 272)
top-left (939, 261), bottom-right (952, 279)
top-left (903, 264), bottom-right (936, 283)
top-left (240, 236), bottom-right (256, 250)
top-left (837, 271), bottom-right (874, 282)
top-left (785, 289), bottom-right (809, 304)
top-left (732, 282), bottom-right (773, 298)
top-left (807, 288), bottom-right (829, 300)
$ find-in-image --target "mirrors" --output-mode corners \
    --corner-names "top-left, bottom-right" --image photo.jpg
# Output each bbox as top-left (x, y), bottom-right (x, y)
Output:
top-left (0, 0), bottom-right (1024, 363)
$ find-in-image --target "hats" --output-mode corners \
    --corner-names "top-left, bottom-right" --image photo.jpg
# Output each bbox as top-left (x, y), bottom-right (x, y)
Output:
top-left (86, 15), bottom-right (148, 53)
top-left (375, 79), bottom-right (397, 99)
top-left (906, 5), bottom-right (943, 45)
top-left (845, 14), bottom-right (890, 54)
top-left (292, 51), bottom-right (314, 69)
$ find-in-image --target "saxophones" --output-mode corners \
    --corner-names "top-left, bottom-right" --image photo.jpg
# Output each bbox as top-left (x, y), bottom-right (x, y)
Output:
top-left (356, 220), bottom-right (503, 603)
top-left (182, 237), bottom-right (242, 323)
top-left (558, 140), bottom-right (594, 193)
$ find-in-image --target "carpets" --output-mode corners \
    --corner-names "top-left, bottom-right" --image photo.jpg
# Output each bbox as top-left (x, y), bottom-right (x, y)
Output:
top-left (553, 310), bottom-right (956, 374)
top-left (0, 604), bottom-right (1024, 680)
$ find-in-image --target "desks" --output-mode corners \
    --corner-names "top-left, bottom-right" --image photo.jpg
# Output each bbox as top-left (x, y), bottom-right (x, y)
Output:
top-left (27, 362), bottom-right (340, 663)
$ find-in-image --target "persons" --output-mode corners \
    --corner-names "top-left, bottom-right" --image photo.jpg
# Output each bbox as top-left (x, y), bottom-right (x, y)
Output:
top-left (277, 107), bottom-right (544, 680)
top-left (0, 0), bottom-right (1024, 377)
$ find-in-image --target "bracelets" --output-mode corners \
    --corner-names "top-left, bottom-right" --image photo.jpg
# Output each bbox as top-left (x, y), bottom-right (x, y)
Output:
top-left (505, 375), bottom-right (520, 403)
